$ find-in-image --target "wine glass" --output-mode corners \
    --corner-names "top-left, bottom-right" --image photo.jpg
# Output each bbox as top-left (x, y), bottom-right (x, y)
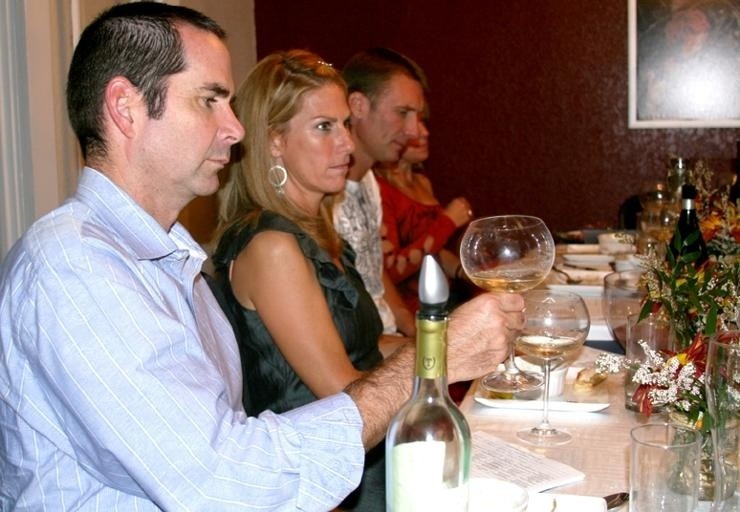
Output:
top-left (460, 215), bottom-right (591, 447)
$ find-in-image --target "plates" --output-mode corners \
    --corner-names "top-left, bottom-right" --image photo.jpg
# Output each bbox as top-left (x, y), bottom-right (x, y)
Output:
top-left (473, 378), bottom-right (610, 413)
top-left (550, 232), bottom-right (647, 342)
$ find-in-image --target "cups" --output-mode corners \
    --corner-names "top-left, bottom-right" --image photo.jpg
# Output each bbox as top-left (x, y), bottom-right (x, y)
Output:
top-left (640, 178), bottom-right (670, 251)
top-left (626, 314), bottom-right (740, 512)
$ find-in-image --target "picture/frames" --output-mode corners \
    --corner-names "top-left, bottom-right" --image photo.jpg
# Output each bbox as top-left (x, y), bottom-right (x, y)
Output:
top-left (626, 0), bottom-right (740, 130)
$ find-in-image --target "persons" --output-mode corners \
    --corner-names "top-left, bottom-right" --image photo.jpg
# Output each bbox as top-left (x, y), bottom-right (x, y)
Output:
top-left (209, 50), bottom-right (385, 512)
top-left (0, 0), bottom-right (525, 512)
top-left (372, 99), bottom-right (474, 316)
top-left (332, 47), bottom-right (428, 358)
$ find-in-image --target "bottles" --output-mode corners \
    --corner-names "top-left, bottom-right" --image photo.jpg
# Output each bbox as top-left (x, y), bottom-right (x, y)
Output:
top-left (385, 254), bottom-right (470, 510)
top-left (664, 157), bottom-right (708, 262)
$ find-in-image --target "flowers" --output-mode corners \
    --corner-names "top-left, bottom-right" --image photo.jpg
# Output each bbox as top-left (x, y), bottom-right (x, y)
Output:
top-left (596, 155), bottom-right (739, 460)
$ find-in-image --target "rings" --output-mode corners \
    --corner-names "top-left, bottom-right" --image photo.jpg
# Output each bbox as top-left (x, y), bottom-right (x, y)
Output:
top-left (468, 210), bottom-right (473, 216)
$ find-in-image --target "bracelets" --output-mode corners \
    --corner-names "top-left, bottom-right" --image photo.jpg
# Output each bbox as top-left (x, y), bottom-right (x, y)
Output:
top-left (455, 264), bottom-right (462, 279)
